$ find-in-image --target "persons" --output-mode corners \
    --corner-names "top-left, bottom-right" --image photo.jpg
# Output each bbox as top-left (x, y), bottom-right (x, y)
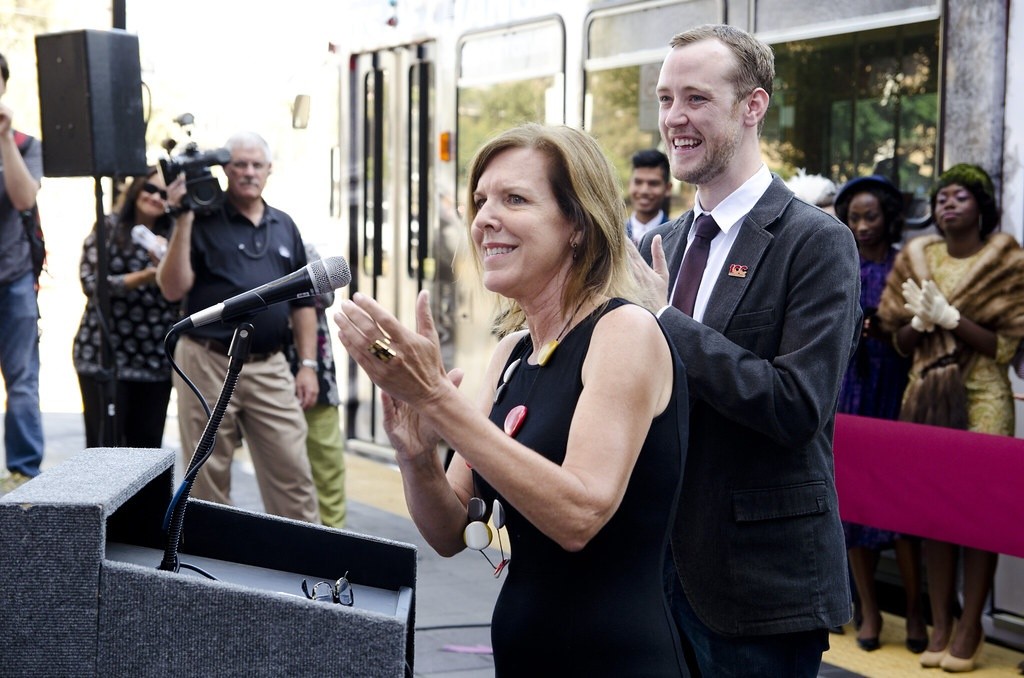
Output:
top-left (335, 124), bottom-right (693, 678)
top-left (72, 128), bottom-right (349, 531)
top-left (609, 24), bottom-right (863, 678)
top-left (0, 54), bottom-right (47, 499)
top-left (620, 149), bottom-right (1024, 672)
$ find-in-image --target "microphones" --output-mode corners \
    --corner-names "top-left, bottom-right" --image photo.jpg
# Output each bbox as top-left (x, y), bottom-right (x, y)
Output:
top-left (174, 257), bottom-right (352, 336)
top-left (162, 139), bottom-right (176, 150)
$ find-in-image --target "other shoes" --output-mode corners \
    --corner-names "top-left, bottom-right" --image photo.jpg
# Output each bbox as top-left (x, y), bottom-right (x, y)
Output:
top-left (829, 625), bottom-right (844, 635)
top-left (853, 609), bottom-right (863, 630)
top-left (2, 471), bottom-right (32, 496)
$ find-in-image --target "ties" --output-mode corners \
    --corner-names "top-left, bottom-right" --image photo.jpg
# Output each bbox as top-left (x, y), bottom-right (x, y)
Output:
top-left (671, 215), bottom-right (721, 321)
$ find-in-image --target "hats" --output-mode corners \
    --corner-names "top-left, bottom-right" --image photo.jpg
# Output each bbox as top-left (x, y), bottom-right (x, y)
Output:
top-left (833, 176), bottom-right (903, 244)
top-left (930, 162), bottom-right (994, 227)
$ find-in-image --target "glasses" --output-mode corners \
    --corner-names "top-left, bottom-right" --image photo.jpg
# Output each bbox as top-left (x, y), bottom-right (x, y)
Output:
top-left (301, 570), bottom-right (354, 606)
top-left (141, 183), bottom-right (167, 200)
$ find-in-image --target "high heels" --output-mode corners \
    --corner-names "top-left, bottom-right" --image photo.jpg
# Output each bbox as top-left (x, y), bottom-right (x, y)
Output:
top-left (906, 616), bottom-right (928, 654)
top-left (939, 623), bottom-right (985, 671)
top-left (858, 614), bottom-right (882, 652)
top-left (920, 619), bottom-right (959, 667)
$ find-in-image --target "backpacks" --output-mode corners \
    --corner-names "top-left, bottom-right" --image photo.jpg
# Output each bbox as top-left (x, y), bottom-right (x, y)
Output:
top-left (12, 131), bottom-right (47, 285)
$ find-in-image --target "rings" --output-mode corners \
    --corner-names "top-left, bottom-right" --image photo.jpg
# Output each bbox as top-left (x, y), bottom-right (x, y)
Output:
top-left (367, 340), bottom-right (397, 364)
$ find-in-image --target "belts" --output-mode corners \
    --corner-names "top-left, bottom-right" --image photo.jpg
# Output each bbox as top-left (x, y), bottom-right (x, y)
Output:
top-left (187, 335), bottom-right (283, 363)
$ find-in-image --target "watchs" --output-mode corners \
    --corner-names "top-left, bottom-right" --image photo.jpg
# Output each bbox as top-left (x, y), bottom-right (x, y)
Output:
top-left (298, 359), bottom-right (319, 373)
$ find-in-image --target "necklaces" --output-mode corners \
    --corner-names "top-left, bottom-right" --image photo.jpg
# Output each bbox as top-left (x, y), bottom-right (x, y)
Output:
top-left (463, 291), bottom-right (591, 577)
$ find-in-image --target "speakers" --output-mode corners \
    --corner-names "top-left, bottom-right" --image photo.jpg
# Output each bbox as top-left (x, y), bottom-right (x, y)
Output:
top-left (35, 29), bottom-right (147, 178)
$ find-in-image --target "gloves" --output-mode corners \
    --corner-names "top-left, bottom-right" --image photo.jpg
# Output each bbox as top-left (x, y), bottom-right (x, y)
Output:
top-left (901, 277), bottom-right (935, 333)
top-left (920, 278), bottom-right (960, 330)
